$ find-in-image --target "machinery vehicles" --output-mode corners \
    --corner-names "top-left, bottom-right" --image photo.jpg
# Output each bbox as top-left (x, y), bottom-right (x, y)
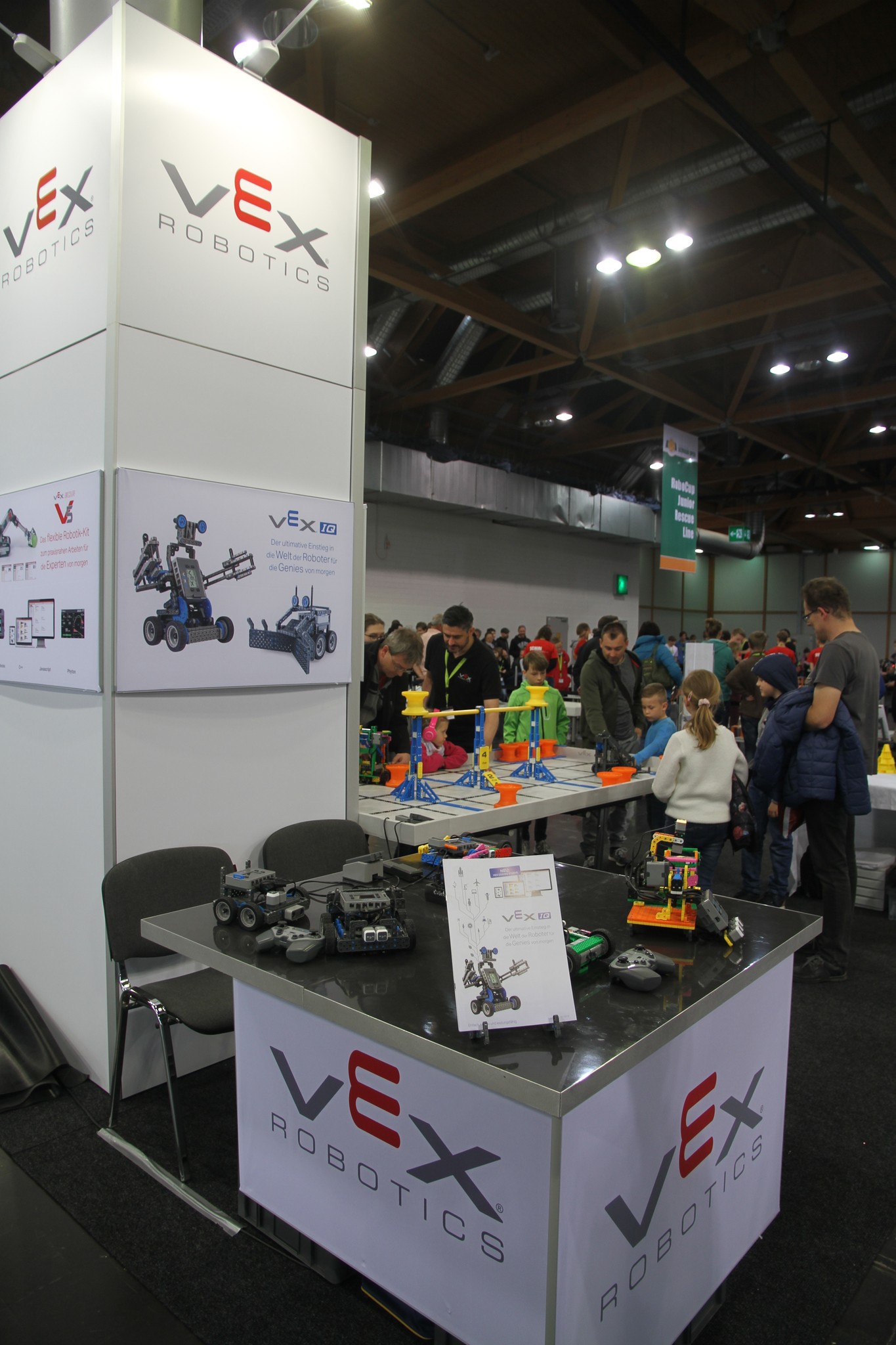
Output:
top-left (418, 831), bottom-right (513, 906)
top-left (562, 920), bottom-right (617, 978)
top-left (358, 724), bottom-right (393, 786)
top-left (213, 859), bottom-right (311, 932)
top-left (319, 886), bottom-right (417, 955)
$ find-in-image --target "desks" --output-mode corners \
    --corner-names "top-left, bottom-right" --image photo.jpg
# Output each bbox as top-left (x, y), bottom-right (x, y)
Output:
top-left (780, 773), bottom-right (896, 898)
top-left (142, 837), bottom-right (825, 1345)
top-left (358, 745), bottom-right (664, 872)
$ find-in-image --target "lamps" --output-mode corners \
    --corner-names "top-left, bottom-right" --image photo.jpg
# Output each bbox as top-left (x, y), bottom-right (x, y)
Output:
top-left (649, 453), bottom-right (664, 470)
top-left (869, 419), bottom-right (887, 433)
top-left (769, 342), bottom-right (849, 375)
top-left (804, 503), bottom-right (844, 518)
top-left (517, 401), bottom-right (575, 430)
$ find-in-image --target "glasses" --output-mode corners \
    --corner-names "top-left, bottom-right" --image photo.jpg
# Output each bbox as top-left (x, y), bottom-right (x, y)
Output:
top-left (389, 648), bottom-right (413, 675)
top-left (365, 634), bottom-right (386, 641)
top-left (802, 608), bottom-right (829, 623)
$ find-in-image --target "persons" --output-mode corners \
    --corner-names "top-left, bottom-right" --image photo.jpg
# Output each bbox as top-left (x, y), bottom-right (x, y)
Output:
top-left (368, 601), bottom-right (896, 907)
top-left (793, 578), bottom-right (881, 985)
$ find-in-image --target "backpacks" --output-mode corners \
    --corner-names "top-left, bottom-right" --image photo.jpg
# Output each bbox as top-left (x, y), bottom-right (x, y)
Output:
top-left (643, 642), bottom-right (675, 689)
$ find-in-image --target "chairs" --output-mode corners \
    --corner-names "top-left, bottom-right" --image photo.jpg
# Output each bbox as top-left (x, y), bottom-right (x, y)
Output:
top-left (263, 818), bottom-right (370, 885)
top-left (95, 846), bottom-right (238, 1241)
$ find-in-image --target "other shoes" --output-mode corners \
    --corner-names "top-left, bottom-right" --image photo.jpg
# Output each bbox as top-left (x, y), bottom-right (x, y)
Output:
top-left (522, 840), bottom-right (533, 856)
top-left (793, 953), bottom-right (847, 982)
top-left (583, 855), bottom-right (595, 867)
top-left (608, 850), bottom-right (631, 867)
top-left (733, 886), bottom-right (760, 902)
top-left (534, 842), bottom-right (556, 859)
top-left (796, 938), bottom-right (819, 954)
top-left (758, 890), bottom-right (785, 909)
